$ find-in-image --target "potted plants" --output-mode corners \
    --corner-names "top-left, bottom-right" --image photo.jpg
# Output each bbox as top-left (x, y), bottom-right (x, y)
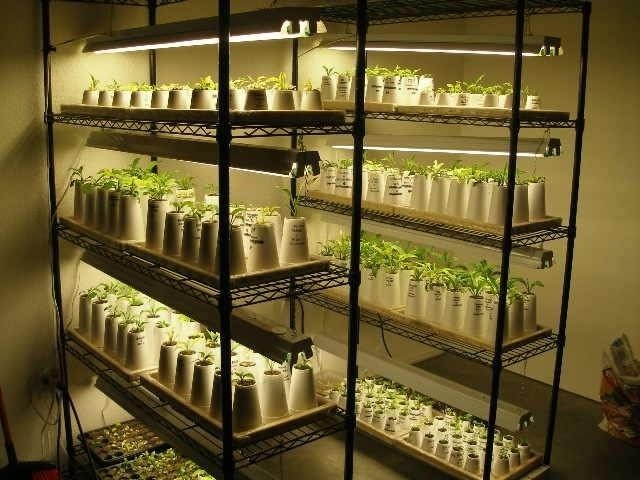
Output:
top-left (78, 278), bottom-right (318, 431)
top-left (66, 156), bottom-right (321, 276)
top-left (328, 373), bottom-right (532, 479)
top-left (322, 149), bottom-right (546, 225)
top-left (81, 71), bottom-right (321, 110)
top-left (313, 229), bottom-right (548, 345)
top-left (322, 64), bottom-right (541, 111)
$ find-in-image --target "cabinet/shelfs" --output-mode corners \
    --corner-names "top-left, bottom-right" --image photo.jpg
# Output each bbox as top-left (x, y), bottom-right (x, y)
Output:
top-left (37, 0), bottom-right (371, 480)
top-left (286, 4), bottom-right (590, 480)
